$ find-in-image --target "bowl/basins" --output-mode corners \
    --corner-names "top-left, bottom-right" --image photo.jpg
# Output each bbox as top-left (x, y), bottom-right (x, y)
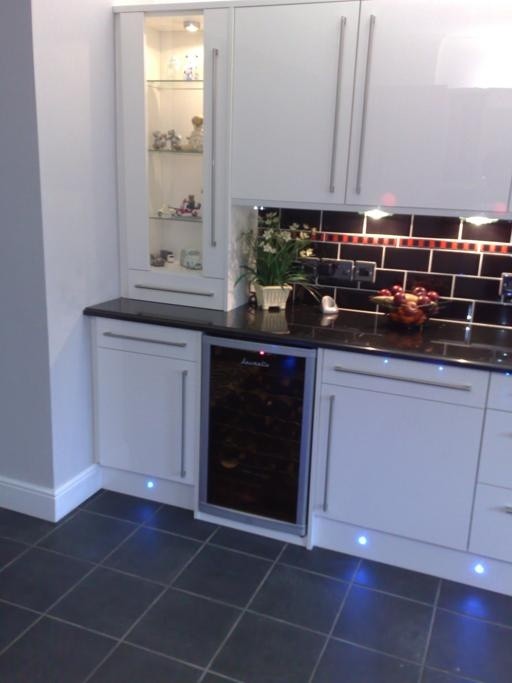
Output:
top-left (375, 288), bottom-right (440, 326)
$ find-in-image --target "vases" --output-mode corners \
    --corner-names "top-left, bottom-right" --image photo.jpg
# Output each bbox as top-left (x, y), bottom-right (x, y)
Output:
top-left (252, 280), bottom-right (292, 310)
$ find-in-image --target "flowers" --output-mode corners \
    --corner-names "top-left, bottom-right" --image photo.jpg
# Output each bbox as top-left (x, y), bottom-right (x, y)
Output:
top-left (234, 210), bottom-right (325, 304)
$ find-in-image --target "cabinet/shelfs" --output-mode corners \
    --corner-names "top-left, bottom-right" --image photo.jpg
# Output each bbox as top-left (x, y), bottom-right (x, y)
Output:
top-left (113, 2), bottom-right (260, 313)
top-left (233, 0), bottom-right (510, 221)
top-left (90, 317), bottom-right (204, 511)
top-left (307, 346), bottom-right (491, 590)
top-left (464, 367), bottom-right (512, 598)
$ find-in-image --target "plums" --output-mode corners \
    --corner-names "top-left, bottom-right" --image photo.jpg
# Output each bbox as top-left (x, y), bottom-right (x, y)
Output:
top-left (379, 284), bottom-right (439, 323)
